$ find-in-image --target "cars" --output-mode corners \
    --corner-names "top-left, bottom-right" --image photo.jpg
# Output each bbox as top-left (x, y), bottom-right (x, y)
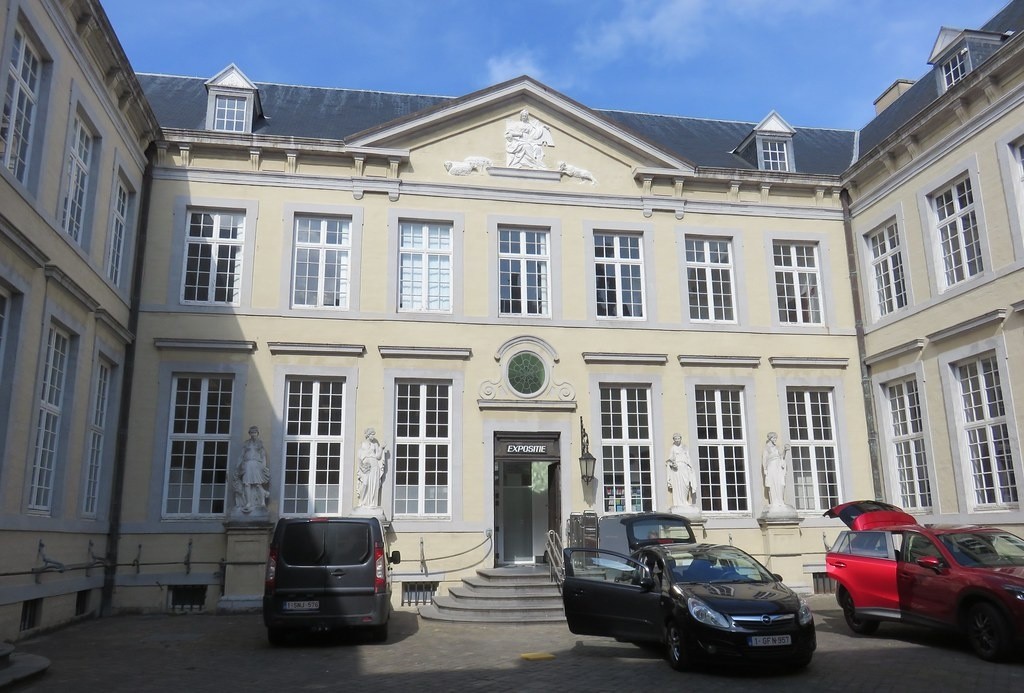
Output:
top-left (822, 499), bottom-right (1024, 663)
top-left (562, 513), bottom-right (817, 674)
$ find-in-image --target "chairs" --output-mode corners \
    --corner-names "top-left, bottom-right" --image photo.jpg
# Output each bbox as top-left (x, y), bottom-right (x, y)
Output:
top-left (656, 557), bottom-right (681, 584)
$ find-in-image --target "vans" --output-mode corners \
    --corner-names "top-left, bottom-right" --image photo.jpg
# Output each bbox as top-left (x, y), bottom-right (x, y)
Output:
top-left (260, 514), bottom-right (401, 647)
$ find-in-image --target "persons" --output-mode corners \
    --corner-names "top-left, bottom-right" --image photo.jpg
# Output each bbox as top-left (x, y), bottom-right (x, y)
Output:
top-left (762, 432), bottom-right (791, 507)
top-left (236, 426), bottom-right (268, 507)
top-left (357, 428), bottom-right (387, 507)
top-left (667, 432), bottom-right (697, 505)
top-left (641, 531), bottom-right (671, 587)
top-left (505, 110), bottom-right (551, 171)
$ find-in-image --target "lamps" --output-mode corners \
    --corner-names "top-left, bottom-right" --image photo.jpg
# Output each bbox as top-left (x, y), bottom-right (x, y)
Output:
top-left (579, 416), bottom-right (596, 485)
